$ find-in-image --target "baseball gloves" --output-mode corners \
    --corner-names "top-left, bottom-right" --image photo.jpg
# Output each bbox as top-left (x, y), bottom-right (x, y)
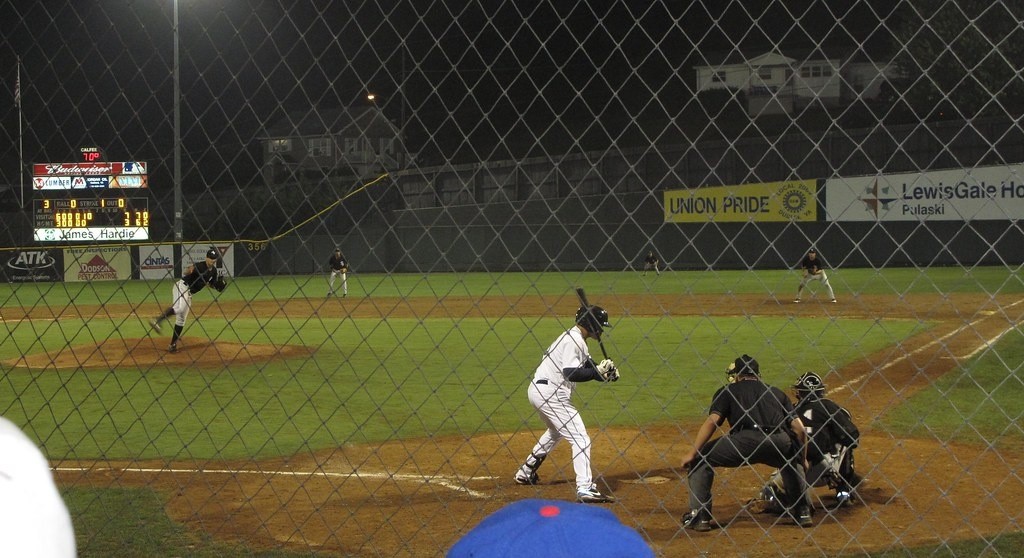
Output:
top-left (214, 275), bottom-right (227, 292)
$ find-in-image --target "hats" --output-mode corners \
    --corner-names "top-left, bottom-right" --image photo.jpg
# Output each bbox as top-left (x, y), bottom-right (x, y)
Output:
top-left (206, 250), bottom-right (220, 259)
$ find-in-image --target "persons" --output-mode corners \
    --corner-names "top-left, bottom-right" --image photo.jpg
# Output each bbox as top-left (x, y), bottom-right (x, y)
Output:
top-left (512, 305), bottom-right (620, 501)
top-left (446, 500), bottom-right (655, 558)
top-left (681, 355), bottom-right (813, 530)
top-left (327, 248), bottom-right (349, 297)
top-left (750, 372), bottom-right (859, 512)
top-left (793, 249), bottom-right (836, 302)
top-left (150, 249), bottom-right (226, 353)
top-left (643, 251), bottom-right (660, 276)
top-left (0, 417), bottom-right (76, 558)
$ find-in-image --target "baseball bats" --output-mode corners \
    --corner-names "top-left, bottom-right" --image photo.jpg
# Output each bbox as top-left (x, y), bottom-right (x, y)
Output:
top-left (574, 285), bottom-right (614, 381)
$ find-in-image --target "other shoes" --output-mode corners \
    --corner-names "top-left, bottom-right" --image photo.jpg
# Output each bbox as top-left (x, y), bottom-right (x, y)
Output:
top-left (831, 298), bottom-right (837, 304)
top-left (148, 320), bottom-right (162, 335)
top-left (794, 298), bottom-right (803, 303)
top-left (166, 344), bottom-right (179, 354)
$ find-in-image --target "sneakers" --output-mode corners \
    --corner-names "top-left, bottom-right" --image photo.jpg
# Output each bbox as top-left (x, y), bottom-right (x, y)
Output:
top-left (794, 505), bottom-right (818, 528)
top-left (681, 509), bottom-right (712, 531)
top-left (512, 475), bottom-right (539, 485)
top-left (838, 487), bottom-right (853, 506)
top-left (576, 488), bottom-right (616, 503)
top-left (748, 487), bottom-right (786, 512)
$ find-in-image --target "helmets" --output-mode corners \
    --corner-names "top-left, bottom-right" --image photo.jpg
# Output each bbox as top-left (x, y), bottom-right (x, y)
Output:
top-left (575, 303), bottom-right (613, 332)
top-left (724, 353), bottom-right (761, 376)
top-left (791, 370), bottom-right (828, 394)
top-left (808, 248), bottom-right (816, 253)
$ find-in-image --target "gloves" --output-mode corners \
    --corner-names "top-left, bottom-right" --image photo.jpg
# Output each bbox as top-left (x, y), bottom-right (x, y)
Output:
top-left (600, 366), bottom-right (620, 383)
top-left (597, 358), bottom-right (615, 375)
